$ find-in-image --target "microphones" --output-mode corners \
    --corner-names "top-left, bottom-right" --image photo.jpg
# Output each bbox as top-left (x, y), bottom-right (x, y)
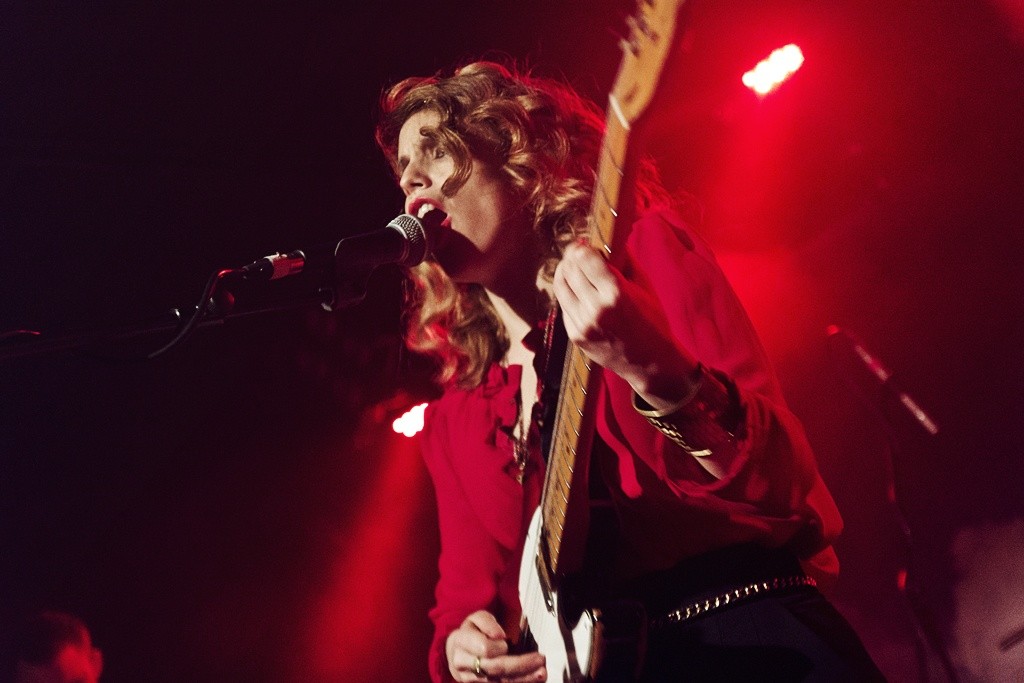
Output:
top-left (835, 331), bottom-right (938, 436)
top-left (230, 215), bottom-right (435, 285)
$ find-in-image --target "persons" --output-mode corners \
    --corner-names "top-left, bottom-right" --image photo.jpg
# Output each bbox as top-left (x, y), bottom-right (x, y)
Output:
top-left (373, 57), bottom-right (889, 683)
top-left (7, 608), bottom-right (104, 683)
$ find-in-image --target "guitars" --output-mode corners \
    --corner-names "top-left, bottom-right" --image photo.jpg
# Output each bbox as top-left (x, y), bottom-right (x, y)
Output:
top-left (502, 0), bottom-right (687, 683)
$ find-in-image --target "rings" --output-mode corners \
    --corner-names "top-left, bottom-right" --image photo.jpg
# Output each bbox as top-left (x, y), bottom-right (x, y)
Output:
top-left (475, 655), bottom-right (483, 678)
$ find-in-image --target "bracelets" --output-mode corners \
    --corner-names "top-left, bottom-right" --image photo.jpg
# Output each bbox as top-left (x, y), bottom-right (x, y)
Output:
top-left (630, 359), bottom-right (742, 458)
